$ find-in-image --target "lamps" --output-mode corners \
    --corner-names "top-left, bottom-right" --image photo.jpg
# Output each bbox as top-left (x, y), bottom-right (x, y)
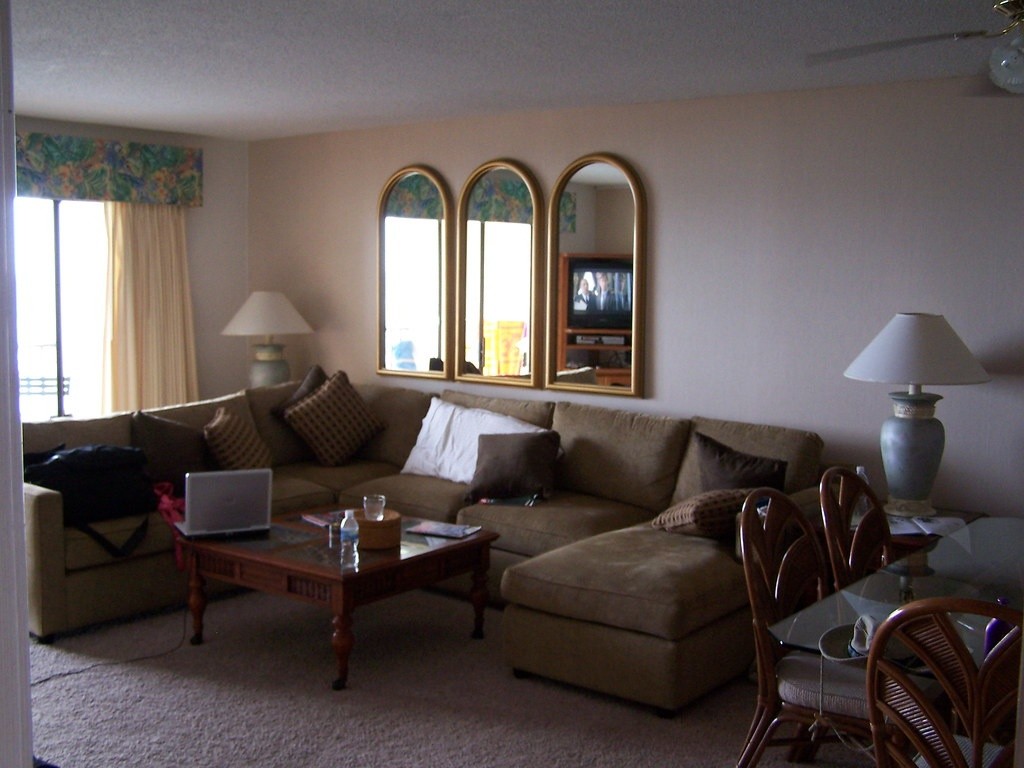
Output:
top-left (218, 290), bottom-right (315, 387)
top-left (843, 312), bottom-right (993, 517)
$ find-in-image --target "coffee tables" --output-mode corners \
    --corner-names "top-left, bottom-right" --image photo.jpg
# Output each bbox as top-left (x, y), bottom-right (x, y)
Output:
top-left (176, 502), bottom-right (500, 693)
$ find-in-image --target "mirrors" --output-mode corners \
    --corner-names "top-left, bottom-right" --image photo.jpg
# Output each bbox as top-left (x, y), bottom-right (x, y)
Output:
top-left (453, 159), bottom-right (544, 388)
top-left (543, 152), bottom-right (646, 400)
top-left (375, 165), bottom-right (453, 381)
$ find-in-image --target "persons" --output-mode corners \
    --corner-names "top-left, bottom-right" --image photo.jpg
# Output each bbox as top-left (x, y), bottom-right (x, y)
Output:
top-left (155, 481), bottom-right (187, 571)
top-left (575, 273), bottom-right (628, 314)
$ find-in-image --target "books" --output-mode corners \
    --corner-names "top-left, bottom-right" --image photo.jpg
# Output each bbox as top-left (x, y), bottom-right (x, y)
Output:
top-left (406, 521), bottom-right (482, 538)
top-left (303, 509), bottom-right (360, 527)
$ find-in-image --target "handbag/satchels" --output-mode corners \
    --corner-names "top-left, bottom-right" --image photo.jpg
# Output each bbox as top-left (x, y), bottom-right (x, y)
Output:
top-left (23, 440), bottom-right (159, 561)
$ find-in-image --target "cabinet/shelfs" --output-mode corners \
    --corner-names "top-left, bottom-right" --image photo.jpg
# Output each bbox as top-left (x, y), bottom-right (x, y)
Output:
top-left (556, 250), bottom-right (634, 388)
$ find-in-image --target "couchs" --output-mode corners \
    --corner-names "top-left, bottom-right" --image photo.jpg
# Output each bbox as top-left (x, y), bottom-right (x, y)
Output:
top-left (20, 379), bottom-right (858, 720)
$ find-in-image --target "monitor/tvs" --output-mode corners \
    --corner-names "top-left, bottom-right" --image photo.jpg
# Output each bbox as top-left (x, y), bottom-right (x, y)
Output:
top-left (569, 267), bottom-right (632, 329)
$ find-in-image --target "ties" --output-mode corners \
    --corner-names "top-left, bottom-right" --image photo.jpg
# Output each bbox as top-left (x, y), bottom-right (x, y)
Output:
top-left (585, 295), bottom-right (589, 304)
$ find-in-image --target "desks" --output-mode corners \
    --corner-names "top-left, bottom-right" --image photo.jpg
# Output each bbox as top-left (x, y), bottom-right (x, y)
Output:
top-left (765, 515), bottom-right (1024, 739)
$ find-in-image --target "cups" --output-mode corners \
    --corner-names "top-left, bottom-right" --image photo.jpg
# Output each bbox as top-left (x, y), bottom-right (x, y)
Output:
top-left (362, 494), bottom-right (386, 522)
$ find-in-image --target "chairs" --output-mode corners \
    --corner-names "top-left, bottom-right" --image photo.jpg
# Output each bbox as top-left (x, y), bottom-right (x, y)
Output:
top-left (734, 468), bottom-right (910, 768)
top-left (865, 597), bottom-right (1024, 768)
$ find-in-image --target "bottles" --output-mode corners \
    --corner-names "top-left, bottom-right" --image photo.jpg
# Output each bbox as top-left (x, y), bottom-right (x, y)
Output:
top-left (340, 509), bottom-right (360, 569)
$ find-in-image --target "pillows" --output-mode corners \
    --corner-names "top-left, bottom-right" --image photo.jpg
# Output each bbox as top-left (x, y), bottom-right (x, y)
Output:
top-left (204, 405), bottom-right (272, 471)
top-left (668, 430), bottom-right (788, 507)
top-left (284, 369), bottom-right (387, 468)
top-left (463, 430), bottom-right (560, 505)
top-left (269, 365), bottom-right (328, 420)
top-left (396, 395), bottom-right (563, 484)
top-left (650, 488), bottom-right (756, 538)
top-left (130, 411), bottom-right (221, 476)
top-left (556, 367), bottom-right (597, 384)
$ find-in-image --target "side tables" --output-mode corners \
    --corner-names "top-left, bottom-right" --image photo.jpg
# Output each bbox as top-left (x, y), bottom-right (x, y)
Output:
top-left (811, 499), bottom-right (984, 559)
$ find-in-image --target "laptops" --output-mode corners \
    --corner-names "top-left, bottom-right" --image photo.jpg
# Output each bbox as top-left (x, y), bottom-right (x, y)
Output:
top-left (173, 468), bottom-right (273, 543)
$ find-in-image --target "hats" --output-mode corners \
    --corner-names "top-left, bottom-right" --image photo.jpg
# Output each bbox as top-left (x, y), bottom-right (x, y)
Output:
top-left (817, 614), bottom-right (974, 674)
